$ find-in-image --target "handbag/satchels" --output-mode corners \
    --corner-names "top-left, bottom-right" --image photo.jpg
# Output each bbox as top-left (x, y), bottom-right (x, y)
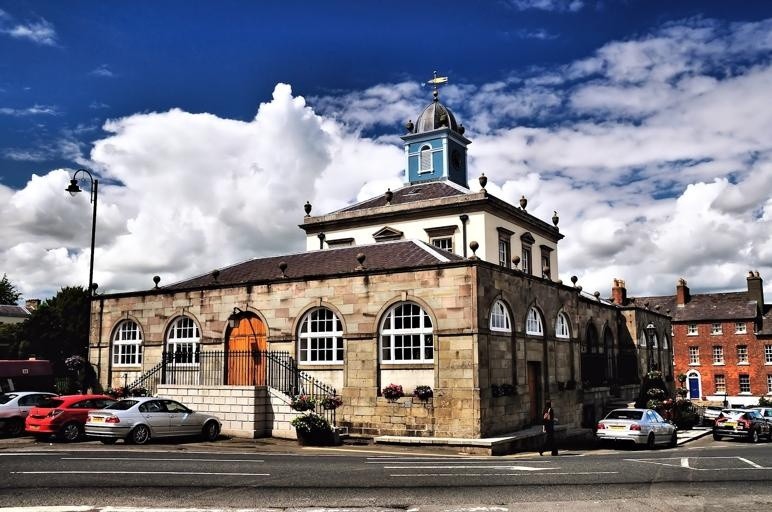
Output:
top-left (543, 413), bottom-right (551, 421)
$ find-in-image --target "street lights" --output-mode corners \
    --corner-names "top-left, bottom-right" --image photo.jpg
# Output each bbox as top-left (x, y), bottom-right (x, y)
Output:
top-left (724, 392), bottom-right (728, 407)
top-left (644, 320), bottom-right (657, 371)
top-left (65, 167), bottom-right (98, 395)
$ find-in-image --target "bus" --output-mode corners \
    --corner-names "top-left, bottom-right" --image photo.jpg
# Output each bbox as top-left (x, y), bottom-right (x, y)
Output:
top-left (0, 359), bottom-right (56, 392)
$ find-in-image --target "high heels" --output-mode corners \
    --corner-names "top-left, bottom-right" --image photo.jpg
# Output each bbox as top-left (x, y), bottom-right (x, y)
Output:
top-left (538, 450), bottom-right (544, 456)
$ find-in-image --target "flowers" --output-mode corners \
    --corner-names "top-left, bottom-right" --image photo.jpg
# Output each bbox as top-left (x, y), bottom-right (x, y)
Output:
top-left (290, 395), bottom-right (315, 411)
top-left (321, 396), bottom-right (343, 409)
top-left (383, 383), bottom-right (404, 401)
top-left (491, 384), bottom-right (515, 397)
top-left (292, 414), bottom-right (332, 432)
top-left (414, 385), bottom-right (433, 402)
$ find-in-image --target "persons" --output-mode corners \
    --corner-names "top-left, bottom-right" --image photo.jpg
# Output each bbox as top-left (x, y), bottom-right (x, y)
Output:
top-left (539, 399), bottom-right (558, 456)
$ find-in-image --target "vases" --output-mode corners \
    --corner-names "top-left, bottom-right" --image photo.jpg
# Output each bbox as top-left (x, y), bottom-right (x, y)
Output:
top-left (296, 427), bottom-right (332, 446)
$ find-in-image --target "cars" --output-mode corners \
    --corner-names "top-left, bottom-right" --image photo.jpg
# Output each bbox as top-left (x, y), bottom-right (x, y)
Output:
top-left (701, 406), bottom-right (772, 444)
top-left (0, 391), bottom-right (222, 445)
top-left (596, 409), bottom-right (677, 450)
